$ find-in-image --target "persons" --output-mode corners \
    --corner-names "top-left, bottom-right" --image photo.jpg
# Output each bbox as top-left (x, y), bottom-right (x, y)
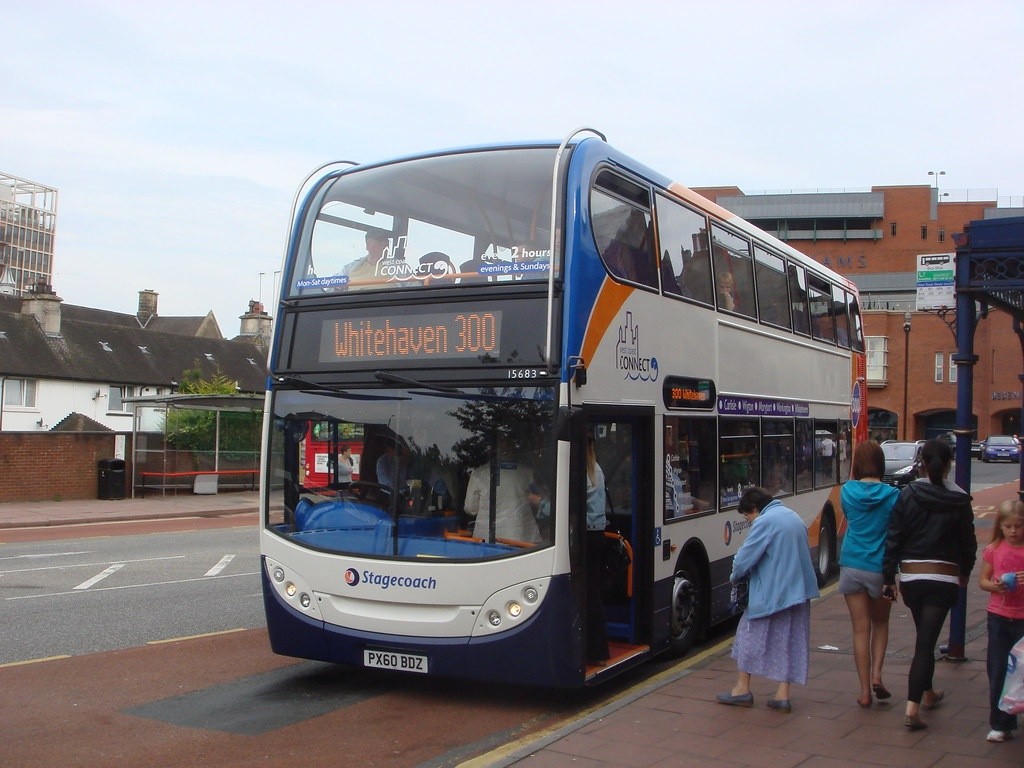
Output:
top-left (516, 241), bottom-right (549, 279)
top-left (881, 441), bottom-right (977, 729)
top-left (338, 443), bottom-right (354, 483)
top-left (804, 431), bottom-right (847, 478)
top-left (837, 441), bottom-right (901, 707)
top-left (526, 430), bottom-right (611, 660)
top-left (1013, 435), bottom-right (1020, 444)
top-left (373, 437), bottom-right (458, 517)
top-left (979, 500), bottom-right (1024, 742)
top-left (337, 227), bottom-right (422, 290)
top-left (463, 435), bottom-right (544, 546)
top-left (715, 486), bottom-right (821, 716)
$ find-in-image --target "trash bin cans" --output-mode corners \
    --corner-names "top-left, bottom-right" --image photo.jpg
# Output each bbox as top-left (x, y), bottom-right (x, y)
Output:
top-left (97, 459), bottom-right (126, 500)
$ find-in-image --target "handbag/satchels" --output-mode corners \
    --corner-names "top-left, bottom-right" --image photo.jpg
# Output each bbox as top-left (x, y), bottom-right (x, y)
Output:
top-left (727, 573), bottom-right (751, 614)
top-left (998, 635), bottom-right (1024, 716)
top-left (606, 538), bottom-right (631, 574)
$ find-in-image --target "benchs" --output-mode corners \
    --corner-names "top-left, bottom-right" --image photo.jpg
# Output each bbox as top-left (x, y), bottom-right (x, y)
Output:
top-left (138, 468), bottom-right (260, 499)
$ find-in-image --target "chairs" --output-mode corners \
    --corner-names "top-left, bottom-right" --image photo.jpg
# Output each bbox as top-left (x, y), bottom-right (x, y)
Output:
top-left (415, 253), bottom-right (456, 284)
top-left (459, 258), bottom-right (488, 283)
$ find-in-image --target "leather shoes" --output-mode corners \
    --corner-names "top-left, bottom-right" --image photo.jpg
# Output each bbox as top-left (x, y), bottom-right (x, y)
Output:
top-left (767, 699), bottom-right (792, 713)
top-left (716, 691), bottom-right (754, 706)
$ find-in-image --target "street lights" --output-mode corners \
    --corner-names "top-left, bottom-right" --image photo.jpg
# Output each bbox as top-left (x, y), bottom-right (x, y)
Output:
top-left (902, 308), bottom-right (911, 441)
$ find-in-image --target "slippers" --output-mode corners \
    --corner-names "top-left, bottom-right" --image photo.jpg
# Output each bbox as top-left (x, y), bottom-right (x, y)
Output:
top-left (873, 685), bottom-right (891, 700)
top-left (857, 697), bottom-right (870, 708)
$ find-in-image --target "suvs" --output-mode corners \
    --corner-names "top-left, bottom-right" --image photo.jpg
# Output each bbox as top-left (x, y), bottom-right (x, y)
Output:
top-left (878, 439), bottom-right (927, 490)
top-left (935, 432), bottom-right (985, 461)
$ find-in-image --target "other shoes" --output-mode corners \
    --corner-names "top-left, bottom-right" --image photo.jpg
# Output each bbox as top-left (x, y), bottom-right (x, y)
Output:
top-left (923, 688), bottom-right (944, 708)
top-left (904, 715), bottom-right (927, 729)
top-left (986, 730), bottom-right (1012, 741)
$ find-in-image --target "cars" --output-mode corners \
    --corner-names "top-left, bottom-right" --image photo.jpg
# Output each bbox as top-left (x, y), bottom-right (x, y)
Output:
top-left (980, 435), bottom-right (1023, 464)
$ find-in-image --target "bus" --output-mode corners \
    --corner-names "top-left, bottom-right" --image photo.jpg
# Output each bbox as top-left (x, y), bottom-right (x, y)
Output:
top-left (295, 421), bottom-right (363, 494)
top-left (259, 126), bottom-right (871, 692)
